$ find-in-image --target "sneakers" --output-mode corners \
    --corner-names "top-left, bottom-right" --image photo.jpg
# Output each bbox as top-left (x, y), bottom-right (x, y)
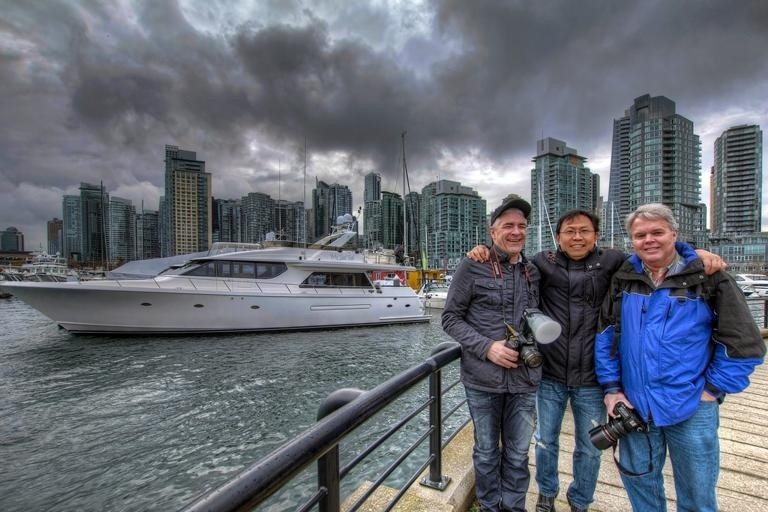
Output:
top-left (566, 493), bottom-right (588, 512)
top-left (537, 488), bottom-right (560, 512)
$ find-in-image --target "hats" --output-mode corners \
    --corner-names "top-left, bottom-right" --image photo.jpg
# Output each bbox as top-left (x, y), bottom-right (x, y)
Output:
top-left (491, 198), bottom-right (531, 226)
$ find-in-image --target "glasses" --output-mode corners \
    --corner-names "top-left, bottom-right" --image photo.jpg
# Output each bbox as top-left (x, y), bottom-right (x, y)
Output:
top-left (558, 231), bottom-right (596, 238)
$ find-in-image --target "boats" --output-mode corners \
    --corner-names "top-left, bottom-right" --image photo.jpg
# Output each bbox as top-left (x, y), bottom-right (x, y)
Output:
top-left (733, 272), bottom-right (768, 301)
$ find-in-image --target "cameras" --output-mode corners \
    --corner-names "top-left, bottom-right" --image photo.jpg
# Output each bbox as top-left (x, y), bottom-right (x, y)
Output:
top-left (505, 308), bottom-right (562, 369)
top-left (588, 402), bottom-right (645, 450)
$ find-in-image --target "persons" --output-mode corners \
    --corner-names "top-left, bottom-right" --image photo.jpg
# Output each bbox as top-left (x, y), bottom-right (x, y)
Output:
top-left (592, 202), bottom-right (767, 512)
top-left (442, 198), bottom-right (541, 511)
top-left (467, 209), bottom-right (729, 512)
